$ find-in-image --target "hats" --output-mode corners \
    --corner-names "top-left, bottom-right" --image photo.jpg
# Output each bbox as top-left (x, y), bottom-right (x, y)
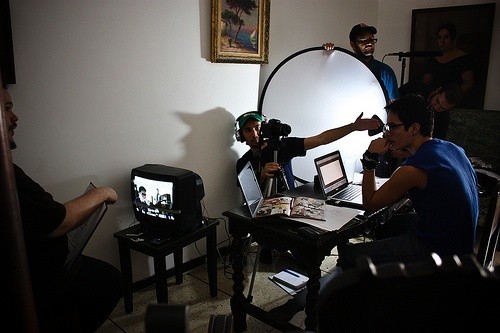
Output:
top-left (240, 112), bottom-right (262, 127)
top-left (350, 24), bottom-right (377, 39)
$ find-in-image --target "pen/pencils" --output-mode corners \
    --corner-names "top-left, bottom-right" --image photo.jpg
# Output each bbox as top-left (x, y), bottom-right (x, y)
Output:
top-left (282, 268), bottom-right (300, 278)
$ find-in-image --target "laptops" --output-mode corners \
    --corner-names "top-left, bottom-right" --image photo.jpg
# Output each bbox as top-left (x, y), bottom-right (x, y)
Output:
top-left (314, 150), bottom-right (364, 206)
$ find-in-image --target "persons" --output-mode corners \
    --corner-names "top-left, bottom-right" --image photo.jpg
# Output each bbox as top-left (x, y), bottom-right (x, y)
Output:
top-left (235, 110), bottom-right (381, 266)
top-left (135, 186), bottom-right (149, 212)
top-left (321, 22), bottom-right (400, 103)
top-left (0, 82), bottom-right (125, 333)
top-left (396, 82), bottom-right (461, 139)
top-left (340, 96), bottom-right (479, 304)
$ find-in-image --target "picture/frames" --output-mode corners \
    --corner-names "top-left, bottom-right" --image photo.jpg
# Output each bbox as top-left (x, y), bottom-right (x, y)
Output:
top-left (409, 3), bottom-right (496, 111)
top-left (210, 0), bottom-right (270, 63)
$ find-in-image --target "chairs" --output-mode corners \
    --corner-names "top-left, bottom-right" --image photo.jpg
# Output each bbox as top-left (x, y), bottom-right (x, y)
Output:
top-left (472, 168), bottom-right (500, 272)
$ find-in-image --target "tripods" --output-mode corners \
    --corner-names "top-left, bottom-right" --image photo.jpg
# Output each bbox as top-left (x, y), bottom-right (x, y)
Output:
top-left (243, 139), bottom-right (291, 304)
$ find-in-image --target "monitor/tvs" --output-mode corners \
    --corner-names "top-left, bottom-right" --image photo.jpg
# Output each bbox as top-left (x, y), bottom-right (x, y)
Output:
top-left (130, 163), bottom-right (205, 235)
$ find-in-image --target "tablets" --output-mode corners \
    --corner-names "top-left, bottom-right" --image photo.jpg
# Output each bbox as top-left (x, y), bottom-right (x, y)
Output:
top-left (238, 161), bottom-right (264, 219)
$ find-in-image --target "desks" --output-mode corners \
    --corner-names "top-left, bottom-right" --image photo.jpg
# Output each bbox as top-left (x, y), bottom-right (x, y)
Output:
top-left (113, 219), bottom-right (221, 314)
top-left (222, 176), bottom-right (396, 333)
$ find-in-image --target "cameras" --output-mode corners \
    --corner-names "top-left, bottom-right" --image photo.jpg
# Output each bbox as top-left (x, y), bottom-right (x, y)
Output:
top-left (261, 118), bottom-right (292, 140)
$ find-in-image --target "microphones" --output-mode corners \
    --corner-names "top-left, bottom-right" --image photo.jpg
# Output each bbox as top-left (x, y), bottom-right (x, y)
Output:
top-left (387, 50), bottom-right (444, 57)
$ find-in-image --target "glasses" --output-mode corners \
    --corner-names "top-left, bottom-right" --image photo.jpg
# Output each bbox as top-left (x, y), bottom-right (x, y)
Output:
top-left (356, 39), bottom-right (377, 45)
top-left (383, 124), bottom-right (405, 133)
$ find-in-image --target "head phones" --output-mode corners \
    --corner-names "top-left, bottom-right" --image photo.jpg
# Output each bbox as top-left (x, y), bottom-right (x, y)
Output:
top-left (234, 111), bottom-right (267, 143)
